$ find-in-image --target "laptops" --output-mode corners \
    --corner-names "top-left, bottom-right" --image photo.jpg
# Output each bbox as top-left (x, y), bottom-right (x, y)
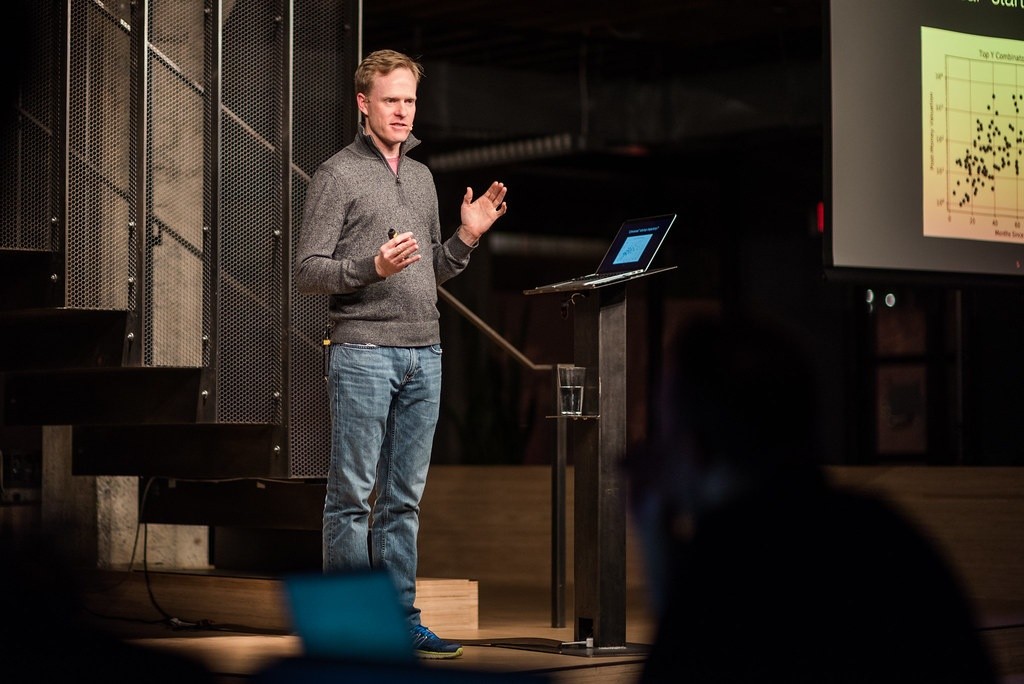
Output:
top-left (534, 214), bottom-right (678, 288)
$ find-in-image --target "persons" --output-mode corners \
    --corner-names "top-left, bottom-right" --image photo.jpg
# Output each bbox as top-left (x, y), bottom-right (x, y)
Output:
top-left (612, 301), bottom-right (1005, 684)
top-left (292, 49), bottom-right (508, 657)
top-left (0, 503), bottom-right (216, 684)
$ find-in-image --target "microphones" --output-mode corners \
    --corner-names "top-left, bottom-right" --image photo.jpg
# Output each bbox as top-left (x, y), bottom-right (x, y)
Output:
top-left (409, 127), bottom-right (413, 131)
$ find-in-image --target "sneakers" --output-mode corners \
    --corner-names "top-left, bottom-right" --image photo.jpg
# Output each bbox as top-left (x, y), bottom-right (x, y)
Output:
top-left (409, 625), bottom-right (463, 659)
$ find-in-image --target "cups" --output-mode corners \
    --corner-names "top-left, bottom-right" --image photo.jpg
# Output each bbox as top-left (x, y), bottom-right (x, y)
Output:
top-left (558, 367), bottom-right (586, 414)
